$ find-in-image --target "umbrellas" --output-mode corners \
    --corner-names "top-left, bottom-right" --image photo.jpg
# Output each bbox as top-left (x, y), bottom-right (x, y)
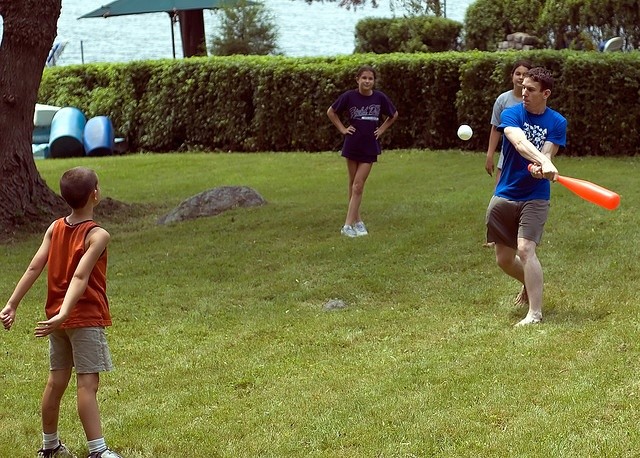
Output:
top-left (75, 0), bottom-right (262, 59)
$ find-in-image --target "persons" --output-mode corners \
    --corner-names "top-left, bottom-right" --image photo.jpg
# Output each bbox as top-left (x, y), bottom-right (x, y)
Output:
top-left (0, 167), bottom-right (118, 458)
top-left (327, 66), bottom-right (398, 239)
top-left (483, 61), bottom-right (531, 248)
top-left (486, 68), bottom-right (568, 328)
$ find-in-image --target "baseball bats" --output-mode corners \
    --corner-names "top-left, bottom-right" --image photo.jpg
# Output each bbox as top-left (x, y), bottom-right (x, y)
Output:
top-left (528, 163), bottom-right (620, 210)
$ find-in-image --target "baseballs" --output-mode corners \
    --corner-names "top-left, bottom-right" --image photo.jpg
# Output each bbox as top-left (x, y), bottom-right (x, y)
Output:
top-left (457, 125), bottom-right (473, 141)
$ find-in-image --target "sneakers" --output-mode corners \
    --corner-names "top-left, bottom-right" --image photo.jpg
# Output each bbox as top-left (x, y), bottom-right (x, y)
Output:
top-left (340, 220), bottom-right (368, 237)
top-left (512, 312), bottom-right (543, 328)
top-left (38, 441), bottom-right (78, 457)
top-left (87, 449), bottom-right (121, 457)
top-left (513, 286), bottom-right (527, 309)
